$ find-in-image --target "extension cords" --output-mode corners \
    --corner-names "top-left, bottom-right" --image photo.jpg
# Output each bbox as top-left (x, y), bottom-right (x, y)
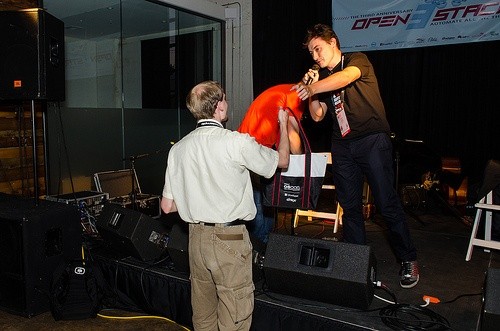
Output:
top-left (423, 296), bottom-right (440, 303)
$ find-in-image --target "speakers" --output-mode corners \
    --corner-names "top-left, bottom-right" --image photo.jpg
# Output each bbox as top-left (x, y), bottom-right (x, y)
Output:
top-left (0, 194), bottom-right (83, 320)
top-left (96, 204), bottom-right (378, 307)
top-left (0, 8), bottom-right (66, 104)
top-left (476, 266), bottom-right (500, 331)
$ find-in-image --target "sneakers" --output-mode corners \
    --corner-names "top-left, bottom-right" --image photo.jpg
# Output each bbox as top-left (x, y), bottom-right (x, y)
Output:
top-left (400, 258), bottom-right (419, 288)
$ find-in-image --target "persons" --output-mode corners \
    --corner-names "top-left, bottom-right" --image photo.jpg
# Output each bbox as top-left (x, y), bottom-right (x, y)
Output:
top-left (237, 82), bottom-right (306, 243)
top-left (159, 78), bottom-right (291, 331)
top-left (289, 22), bottom-right (421, 290)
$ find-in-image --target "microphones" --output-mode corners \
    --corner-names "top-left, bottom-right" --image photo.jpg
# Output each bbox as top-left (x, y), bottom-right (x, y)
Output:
top-left (306, 64), bottom-right (320, 86)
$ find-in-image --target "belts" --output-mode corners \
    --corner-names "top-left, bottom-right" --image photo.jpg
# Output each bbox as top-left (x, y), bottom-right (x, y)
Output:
top-left (195, 219), bottom-right (244, 226)
